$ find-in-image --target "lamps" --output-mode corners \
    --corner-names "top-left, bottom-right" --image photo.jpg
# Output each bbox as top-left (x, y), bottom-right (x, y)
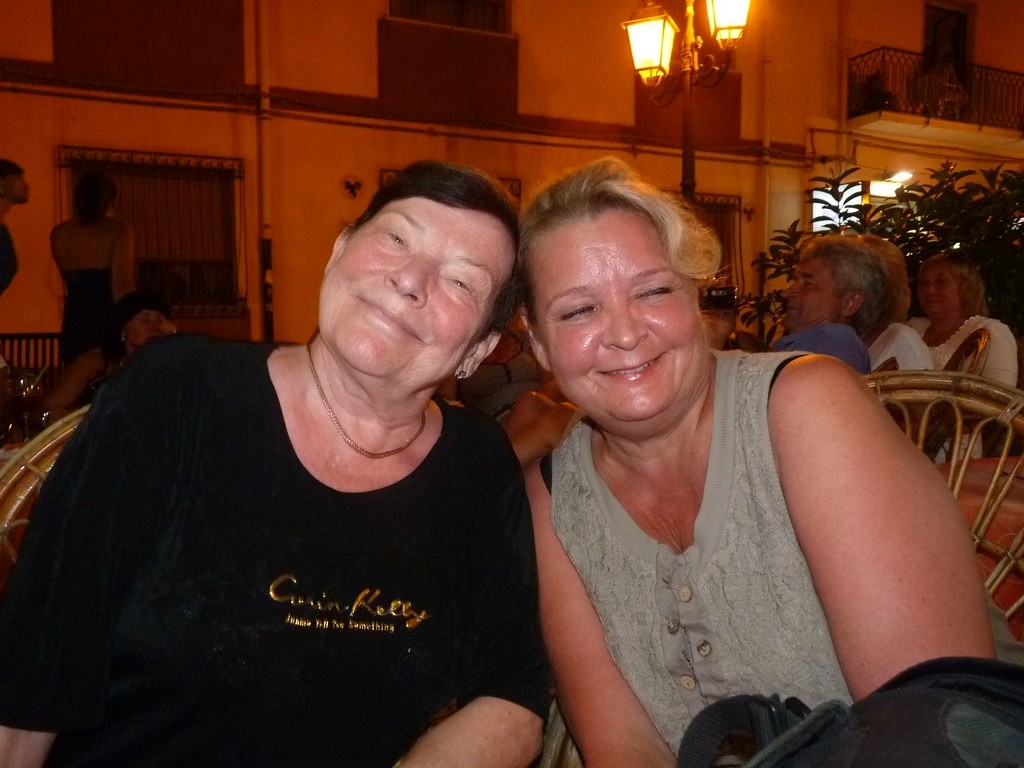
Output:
top-left (885, 171), bottom-right (913, 186)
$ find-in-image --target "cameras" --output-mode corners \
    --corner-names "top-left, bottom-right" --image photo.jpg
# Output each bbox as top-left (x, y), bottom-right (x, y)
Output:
top-left (702, 286), bottom-right (737, 310)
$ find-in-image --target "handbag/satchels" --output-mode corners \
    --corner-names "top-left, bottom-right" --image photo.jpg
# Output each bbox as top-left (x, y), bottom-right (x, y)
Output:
top-left (675, 654), bottom-right (1024, 768)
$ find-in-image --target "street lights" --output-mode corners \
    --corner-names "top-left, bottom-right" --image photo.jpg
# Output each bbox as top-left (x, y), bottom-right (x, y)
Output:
top-left (622, 0), bottom-right (755, 214)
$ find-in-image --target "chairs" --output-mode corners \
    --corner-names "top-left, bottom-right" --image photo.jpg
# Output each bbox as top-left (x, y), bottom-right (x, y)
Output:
top-left (539, 327), bottom-right (1022, 768)
top-left (0, 402), bottom-right (93, 596)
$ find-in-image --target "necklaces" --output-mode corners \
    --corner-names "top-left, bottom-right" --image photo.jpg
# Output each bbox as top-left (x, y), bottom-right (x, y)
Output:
top-left (306, 340), bottom-right (426, 459)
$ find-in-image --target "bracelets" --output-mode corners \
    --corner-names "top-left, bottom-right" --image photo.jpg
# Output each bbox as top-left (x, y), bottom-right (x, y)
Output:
top-left (167, 329), bottom-right (176, 334)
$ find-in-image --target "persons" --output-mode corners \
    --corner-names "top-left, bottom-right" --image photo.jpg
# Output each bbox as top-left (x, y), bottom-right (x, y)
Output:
top-left (866, 235), bottom-right (933, 424)
top-left (770, 239), bottom-right (882, 376)
top-left (519, 158), bottom-right (996, 768)
top-left (918, 256), bottom-right (1018, 463)
top-left (29, 290), bottom-right (178, 427)
top-left (436, 315), bottom-right (556, 422)
top-left (0, 158), bottom-right (29, 295)
top-left (700, 286), bottom-right (771, 353)
top-left (0, 160), bottom-right (546, 768)
top-left (49, 171), bottom-right (136, 368)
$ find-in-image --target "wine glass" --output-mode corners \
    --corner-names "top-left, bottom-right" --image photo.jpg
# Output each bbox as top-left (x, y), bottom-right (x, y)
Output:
top-left (5, 371), bottom-right (44, 445)
